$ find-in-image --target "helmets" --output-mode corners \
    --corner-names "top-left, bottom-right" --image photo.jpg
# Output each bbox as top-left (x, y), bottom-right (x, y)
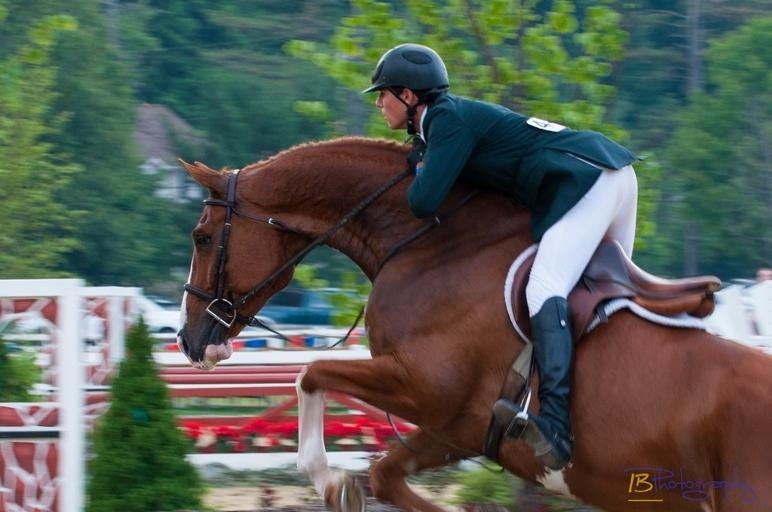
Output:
top-left (359, 43), bottom-right (450, 95)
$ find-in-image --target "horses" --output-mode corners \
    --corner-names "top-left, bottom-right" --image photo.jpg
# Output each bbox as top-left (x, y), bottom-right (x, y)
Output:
top-left (175, 134), bottom-right (772, 511)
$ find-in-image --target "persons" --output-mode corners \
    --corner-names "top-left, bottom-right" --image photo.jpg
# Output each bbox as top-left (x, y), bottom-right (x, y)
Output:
top-left (746, 266), bottom-right (772, 290)
top-left (360, 41), bottom-right (640, 472)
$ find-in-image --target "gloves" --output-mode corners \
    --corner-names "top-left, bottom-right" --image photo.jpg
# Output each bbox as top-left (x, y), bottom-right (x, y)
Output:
top-left (406, 137), bottom-right (428, 171)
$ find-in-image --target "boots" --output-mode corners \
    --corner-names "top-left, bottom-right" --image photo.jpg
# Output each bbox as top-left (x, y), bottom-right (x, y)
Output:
top-left (490, 295), bottom-right (574, 473)
